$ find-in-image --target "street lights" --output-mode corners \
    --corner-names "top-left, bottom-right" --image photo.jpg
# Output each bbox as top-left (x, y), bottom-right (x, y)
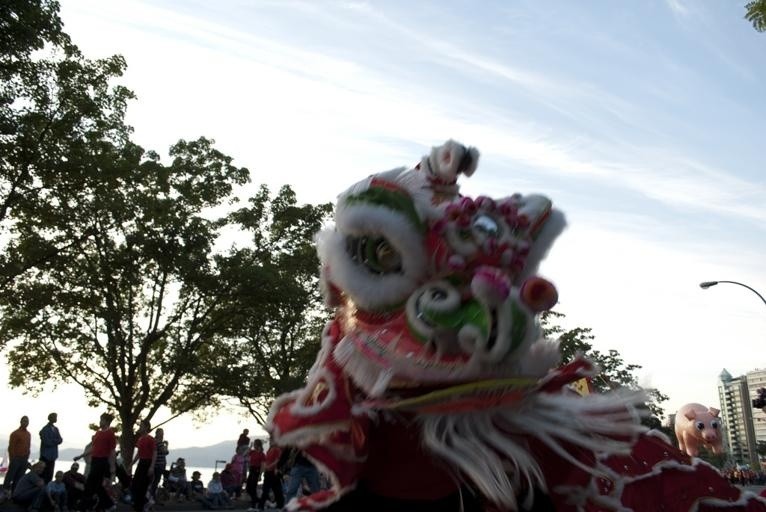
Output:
top-left (699, 281), bottom-right (766, 304)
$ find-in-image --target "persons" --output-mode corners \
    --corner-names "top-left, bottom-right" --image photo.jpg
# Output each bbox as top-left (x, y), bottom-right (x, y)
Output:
top-left (721, 466), bottom-right (766, 486)
top-left (1, 412), bottom-right (321, 510)
